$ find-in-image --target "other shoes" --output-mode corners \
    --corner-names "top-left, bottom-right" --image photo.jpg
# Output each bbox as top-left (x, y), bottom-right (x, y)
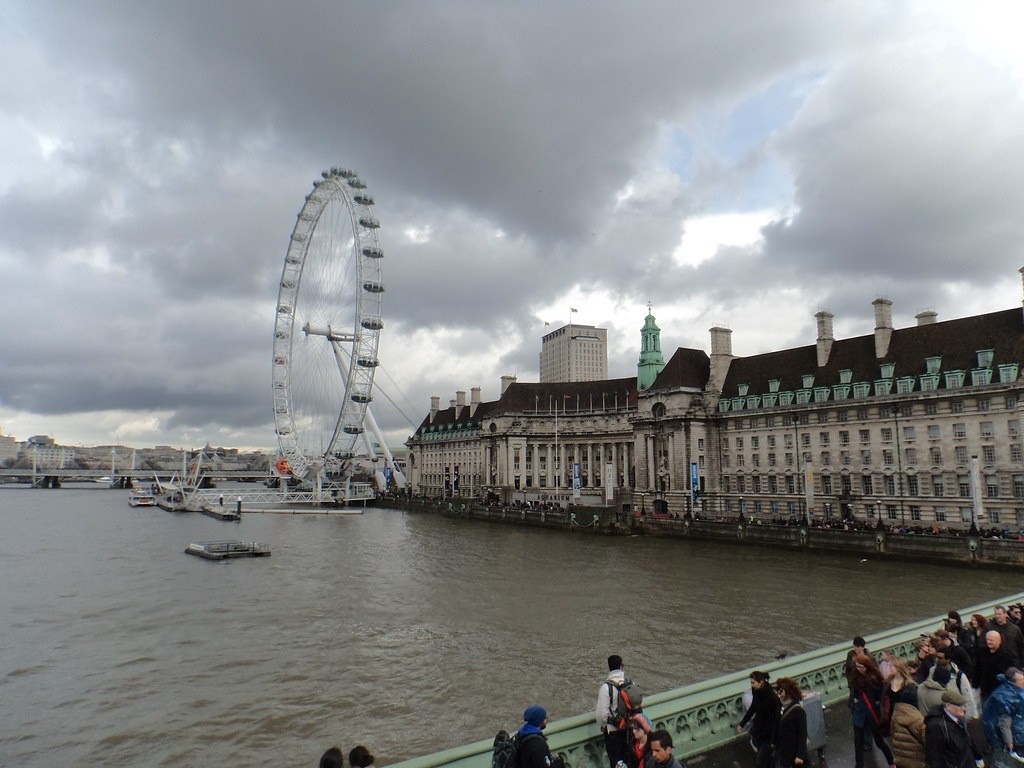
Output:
top-left (889, 763), bottom-right (896, 768)
top-left (864, 744), bottom-right (873, 750)
top-left (975, 759), bottom-right (985, 768)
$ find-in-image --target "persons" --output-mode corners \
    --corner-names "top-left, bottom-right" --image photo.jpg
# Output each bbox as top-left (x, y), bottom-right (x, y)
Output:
top-left (319, 747), bottom-right (343, 768)
top-left (491, 500), bottom-right (564, 511)
top-left (615, 713), bottom-right (652, 768)
top-left (514, 705), bottom-right (564, 768)
top-left (597, 655), bottom-right (644, 768)
top-left (771, 515), bottom-right (1024, 541)
top-left (646, 729), bottom-right (683, 768)
top-left (348, 746), bottom-right (375, 768)
top-left (776, 679), bottom-right (811, 768)
top-left (799, 684), bottom-right (828, 768)
top-left (737, 671), bottom-right (783, 768)
top-left (845, 602), bottom-right (1024, 768)
top-left (742, 672), bottom-right (771, 752)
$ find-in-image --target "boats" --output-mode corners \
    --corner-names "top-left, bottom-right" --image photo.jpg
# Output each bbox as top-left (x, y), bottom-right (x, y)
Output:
top-left (153, 482), bottom-right (204, 513)
top-left (124, 478), bottom-right (139, 483)
top-left (128, 485), bottom-right (156, 507)
top-left (96, 477), bottom-right (112, 483)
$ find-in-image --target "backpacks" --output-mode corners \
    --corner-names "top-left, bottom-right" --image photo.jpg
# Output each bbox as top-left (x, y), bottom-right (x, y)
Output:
top-left (607, 679), bottom-right (643, 729)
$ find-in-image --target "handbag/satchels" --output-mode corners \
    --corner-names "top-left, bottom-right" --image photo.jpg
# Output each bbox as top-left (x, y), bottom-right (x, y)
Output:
top-left (877, 692), bottom-right (890, 736)
top-left (807, 738), bottom-right (812, 751)
top-left (748, 726), bottom-right (760, 752)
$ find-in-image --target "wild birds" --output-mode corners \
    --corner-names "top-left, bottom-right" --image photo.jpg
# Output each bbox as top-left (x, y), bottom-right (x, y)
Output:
top-left (774, 646), bottom-right (789, 663)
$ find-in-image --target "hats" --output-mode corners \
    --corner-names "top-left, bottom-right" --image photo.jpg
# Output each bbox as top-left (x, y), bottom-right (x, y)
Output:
top-left (942, 691), bottom-right (967, 705)
top-left (524, 707), bottom-right (547, 721)
top-left (920, 632), bottom-right (935, 638)
top-left (630, 713), bottom-right (653, 733)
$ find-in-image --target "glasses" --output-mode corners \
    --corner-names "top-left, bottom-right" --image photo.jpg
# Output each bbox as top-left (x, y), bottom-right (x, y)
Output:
top-left (936, 655), bottom-right (945, 659)
top-left (855, 666), bottom-right (866, 670)
top-left (1015, 611), bottom-right (1021, 614)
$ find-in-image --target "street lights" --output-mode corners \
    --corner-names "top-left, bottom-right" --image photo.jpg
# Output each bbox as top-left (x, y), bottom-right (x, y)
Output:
top-left (970, 504), bottom-right (978, 534)
top-left (524, 490), bottom-right (526, 507)
top-left (800, 499), bottom-right (807, 525)
top-left (877, 500), bottom-right (883, 529)
top-left (739, 497), bottom-right (744, 521)
top-left (848, 504), bottom-right (853, 529)
top-left (640, 493), bottom-right (646, 515)
top-left (825, 503), bottom-right (830, 528)
